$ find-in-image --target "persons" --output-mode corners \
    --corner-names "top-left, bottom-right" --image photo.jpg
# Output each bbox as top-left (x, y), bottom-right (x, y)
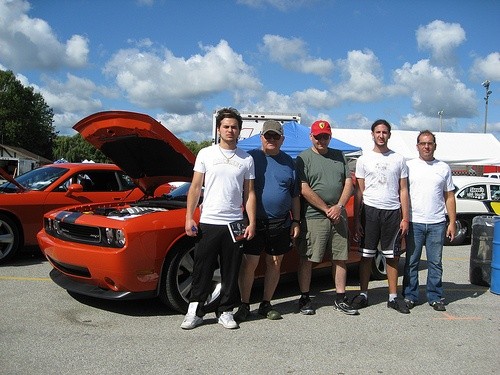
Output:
top-left (180, 107), bottom-right (257, 331)
top-left (351, 120), bottom-right (410, 314)
top-left (234, 119), bottom-right (302, 320)
top-left (294, 120), bottom-right (359, 315)
top-left (403, 130), bottom-right (456, 309)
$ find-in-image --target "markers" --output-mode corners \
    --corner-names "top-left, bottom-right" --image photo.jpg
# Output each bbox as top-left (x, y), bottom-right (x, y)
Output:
top-left (192, 227), bottom-right (196, 231)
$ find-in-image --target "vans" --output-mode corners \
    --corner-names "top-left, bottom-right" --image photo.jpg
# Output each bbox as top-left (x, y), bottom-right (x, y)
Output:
top-left (483, 173), bottom-right (500, 179)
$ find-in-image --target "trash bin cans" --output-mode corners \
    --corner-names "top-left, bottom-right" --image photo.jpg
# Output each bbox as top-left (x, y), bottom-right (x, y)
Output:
top-left (469, 216), bottom-right (494, 287)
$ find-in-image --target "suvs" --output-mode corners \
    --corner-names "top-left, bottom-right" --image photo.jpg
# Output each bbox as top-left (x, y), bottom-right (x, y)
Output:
top-left (35, 110), bottom-right (363, 316)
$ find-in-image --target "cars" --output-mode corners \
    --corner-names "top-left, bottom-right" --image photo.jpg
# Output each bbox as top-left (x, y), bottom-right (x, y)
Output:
top-left (0, 162), bottom-right (177, 264)
top-left (444, 175), bottom-right (500, 247)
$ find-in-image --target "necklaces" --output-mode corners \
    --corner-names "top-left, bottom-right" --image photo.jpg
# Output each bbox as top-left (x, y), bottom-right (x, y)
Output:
top-left (219, 145), bottom-right (237, 163)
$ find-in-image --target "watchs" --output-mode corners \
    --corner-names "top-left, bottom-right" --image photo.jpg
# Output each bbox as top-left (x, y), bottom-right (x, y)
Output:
top-left (292, 219), bottom-right (302, 225)
top-left (337, 203), bottom-right (344, 208)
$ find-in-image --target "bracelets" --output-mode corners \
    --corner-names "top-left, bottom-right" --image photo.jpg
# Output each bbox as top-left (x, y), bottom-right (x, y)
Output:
top-left (401, 219), bottom-right (409, 223)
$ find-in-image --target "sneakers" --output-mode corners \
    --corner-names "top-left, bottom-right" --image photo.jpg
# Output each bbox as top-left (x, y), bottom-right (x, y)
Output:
top-left (258, 303), bottom-right (281, 320)
top-left (218, 311), bottom-right (239, 329)
top-left (430, 301), bottom-right (446, 311)
top-left (234, 304), bottom-right (250, 322)
top-left (387, 297), bottom-right (410, 313)
top-left (297, 298), bottom-right (314, 315)
top-left (403, 298), bottom-right (417, 308)
top-left (349, 294), bottom-right (368, 309)
top-left (180, 301), bottom-right (204, 329)
top-left (333, 295), bottom-right (358, 314)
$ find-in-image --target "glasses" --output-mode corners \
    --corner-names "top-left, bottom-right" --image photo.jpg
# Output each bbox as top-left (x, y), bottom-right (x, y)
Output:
top-left (313, 134), bottom-right (329, 140)
top-left (417, 141), bottom-right (436, 146)
top-left (261, 133), bottom-right (281, 141)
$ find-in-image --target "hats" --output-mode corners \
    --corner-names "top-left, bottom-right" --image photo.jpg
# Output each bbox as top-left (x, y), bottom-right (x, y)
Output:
top-left (261, 120), bottom-right (282, 135)
top-left (311, 120), bottom-right (332, 136)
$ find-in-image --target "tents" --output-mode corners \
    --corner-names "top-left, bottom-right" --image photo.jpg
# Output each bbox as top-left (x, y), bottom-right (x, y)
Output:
top-left (211, 113), bottom-right (500, 177)
top-left (237, 121), bottom-right (361, 158)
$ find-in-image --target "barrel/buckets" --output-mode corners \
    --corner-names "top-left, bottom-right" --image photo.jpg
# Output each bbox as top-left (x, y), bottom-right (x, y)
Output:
top-left (469, 215), bottom-right (500, 295)
